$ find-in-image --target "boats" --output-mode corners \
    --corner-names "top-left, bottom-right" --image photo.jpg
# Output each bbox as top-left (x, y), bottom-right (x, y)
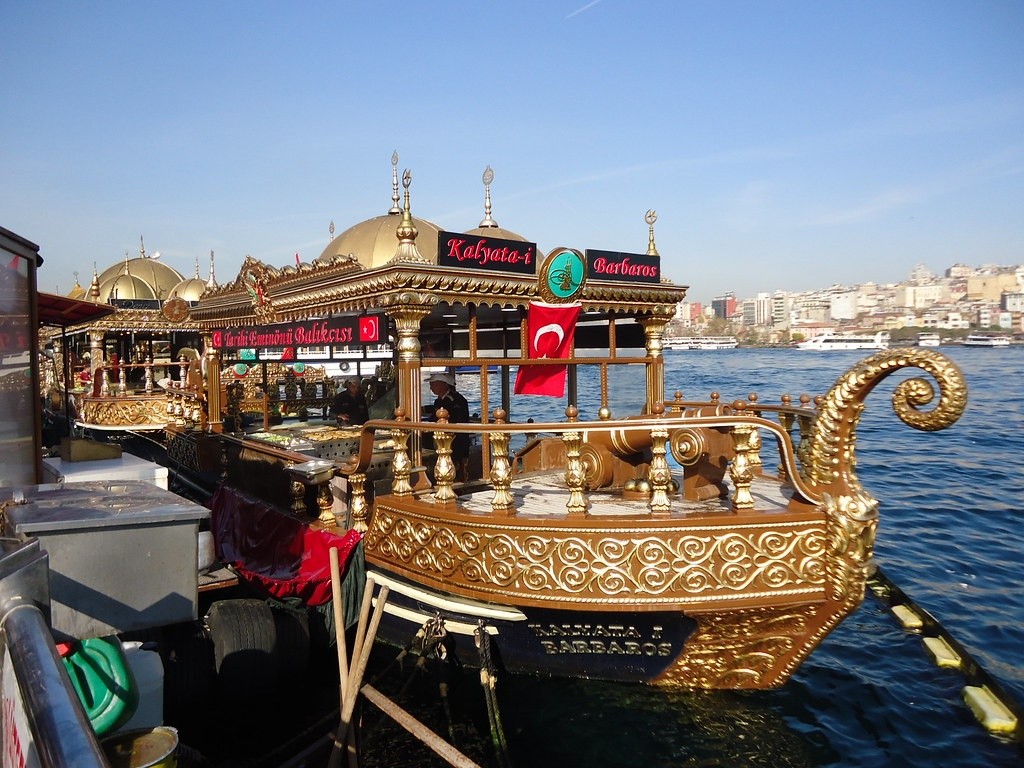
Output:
top-left (660, 336), bottom-right (739, 350)
top-left (162, 150), bottom-right (968, 691)
top-left (36, 237), bottom-right (187, 430)
top-left (912, 332), bottom-right (940, 347)
top-left (794, 331), bottom-right (891, 350)
top-left (960, 331), bottom-right (1011, 347)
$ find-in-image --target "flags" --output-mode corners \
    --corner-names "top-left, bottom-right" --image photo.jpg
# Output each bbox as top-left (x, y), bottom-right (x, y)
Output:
top-left (514, 301), bottom-right (582, 397)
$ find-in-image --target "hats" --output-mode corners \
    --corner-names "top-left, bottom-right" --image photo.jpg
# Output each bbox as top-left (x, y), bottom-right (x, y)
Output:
top-left (447, 376), bottom-right (456, 386)
top-left (348, 375), bottom-right (362, 383)
top-left (424, 374), bottom-right (448, 382)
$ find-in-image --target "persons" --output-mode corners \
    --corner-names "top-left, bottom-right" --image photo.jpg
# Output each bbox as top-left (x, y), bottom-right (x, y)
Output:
top-left (77, 365), bottom-right (91, 387)
top-left (329, 376), bottom-right (369, 426)
top-left (130, 362), bottom-right (145, 383)
top-left (420, 374), bottom-right (471, 465)
